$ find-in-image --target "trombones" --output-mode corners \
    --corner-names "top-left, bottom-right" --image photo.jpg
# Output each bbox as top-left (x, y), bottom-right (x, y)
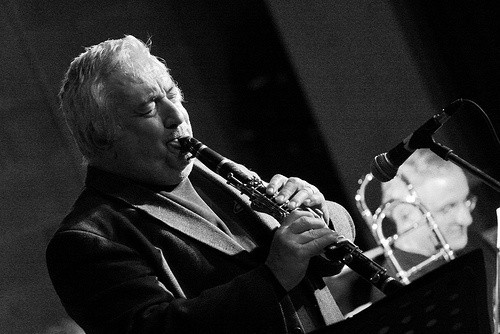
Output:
top-left (355, 165), bottom-right (457, 285)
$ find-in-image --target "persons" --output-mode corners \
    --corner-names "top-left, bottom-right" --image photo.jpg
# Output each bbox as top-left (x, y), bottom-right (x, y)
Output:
top-left (46, 35), bottom-right (356, 334)
top-left (370, 148), bottom-right (500, 334)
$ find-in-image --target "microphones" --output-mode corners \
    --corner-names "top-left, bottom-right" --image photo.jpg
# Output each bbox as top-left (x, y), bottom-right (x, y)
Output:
top-left (371, 99), bottom-right (465, 182)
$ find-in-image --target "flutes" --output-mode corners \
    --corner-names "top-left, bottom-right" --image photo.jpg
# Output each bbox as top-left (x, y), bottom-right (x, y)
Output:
top-left (179, 131), bottom-right (405, 299)
